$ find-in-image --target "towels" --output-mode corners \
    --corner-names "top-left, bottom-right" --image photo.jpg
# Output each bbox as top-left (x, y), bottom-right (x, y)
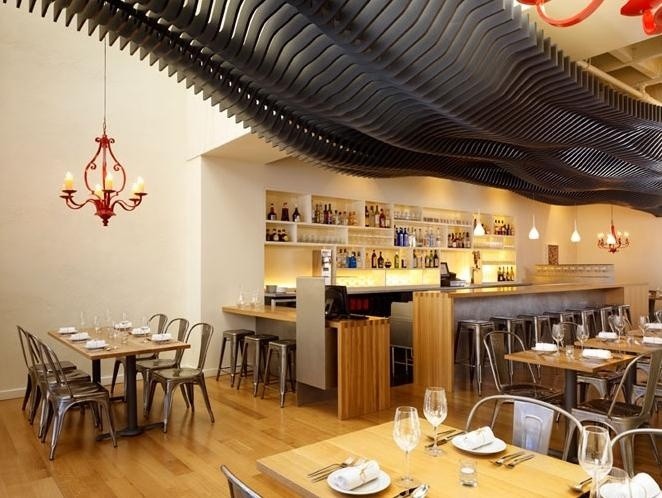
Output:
top-left (615, 473), bottom-right (660, 498)
top-left (333, 460), bottom-right (379, 489)
top-left (462, 427), bottom-right (494, 448)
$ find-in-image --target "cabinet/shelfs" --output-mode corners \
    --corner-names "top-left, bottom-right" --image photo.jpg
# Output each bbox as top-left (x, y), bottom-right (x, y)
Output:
top-left (263, 186), bottom-right (517, 287)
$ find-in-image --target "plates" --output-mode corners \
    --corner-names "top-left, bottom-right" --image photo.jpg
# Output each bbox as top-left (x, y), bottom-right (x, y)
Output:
top-left (582, 355), bottom-right (614, 362)
top-left (452, 435), bottom-right (506, 456)
top-left (327, 467), bottom-right (391, 495)
top-left (531, 347), bottom-right (557, 354)
top-left (58, 325), bottom-right (172, 349)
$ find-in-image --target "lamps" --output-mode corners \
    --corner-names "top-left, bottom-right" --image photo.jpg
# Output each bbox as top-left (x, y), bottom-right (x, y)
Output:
top-left (528, 192), bottom-right (539, 240)
top-left (59, 37), bottom-right (148, 227)
top-left (570, 198), bottom-right (581, 242)
top-left (597, 201), bottom-right (631, 255)
top-left (473, 185), bottom-right (486, 237)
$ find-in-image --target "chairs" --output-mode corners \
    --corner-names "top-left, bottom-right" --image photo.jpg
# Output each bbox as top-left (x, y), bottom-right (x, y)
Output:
top-left (465, 394), bottom-right (583, 465)
top-left (220, 464), bottom-right (262, 498)
top-left (483, 330), bottom-right (662, 478)
top-left (17, 313), bottom-right (215, 460)
top-left (595, 429), bottom-right (662, 498)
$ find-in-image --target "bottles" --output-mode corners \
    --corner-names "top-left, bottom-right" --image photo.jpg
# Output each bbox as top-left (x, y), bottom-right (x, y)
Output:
top-left (494, 220), bottom-right (514, 235)
top-left (365, 205), bottom-right (391, 228)
top-left (312, 200), bottom-right (357, 225)
top-left (266, 229), bottom-right (289, 242)
top-left (448, 232), bottom-right (470, 248)
top-left (394, 225), bottom-right (441, 247)
top-left (413, 249), bottom-right (438, 268)
top-left (497, 266), bottom-right (514, 281)
top-left (337, 248), bottom-right (408, 268)
top-left (268, 201), bottom-right (301, 223)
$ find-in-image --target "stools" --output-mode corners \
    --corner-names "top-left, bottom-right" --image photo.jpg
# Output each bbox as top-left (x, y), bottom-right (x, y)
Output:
top-left (216, 329), bottom-right (255, 388)
top-left (260, 339), bottom-right (295, 408)
top-left (454, 304), bottom-right (631, 397)
top-left (237, 334), bottom-right (279, 397)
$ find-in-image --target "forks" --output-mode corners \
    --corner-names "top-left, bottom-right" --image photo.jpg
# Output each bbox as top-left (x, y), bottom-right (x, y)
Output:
top-left (306, 454), bottom-right (355, 478)
top-left (489, 451), bottom-right (525, 465)
top-left (504, 455), bottom-right (535, 468)
top-left (312, 458), bottom-right (366, 483)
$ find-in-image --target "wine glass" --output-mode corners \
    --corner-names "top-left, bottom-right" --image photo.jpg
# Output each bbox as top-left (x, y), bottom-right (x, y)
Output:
top-left (423, 387), bottom-right (448, 457)
top-left (552, 324), bottom-right (565, 356)
top-left (578, 426), bottom-right (614, 498)
top-left (608, 315), bottom-right (650, 343)
top-left (79, 308), bottom-right (149, 349)
top-left (597, 467), bottom-right (632, 498)
top-left (576, 325), bottom-right (589, 360)
top-left (613, 331), bottom-right (624, 359)
top-left (393, 406), bottom-right (420, 488)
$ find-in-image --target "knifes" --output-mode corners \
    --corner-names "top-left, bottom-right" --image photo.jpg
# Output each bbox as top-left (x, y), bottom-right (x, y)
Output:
top-left (426, 432), bottom-right (465, 447)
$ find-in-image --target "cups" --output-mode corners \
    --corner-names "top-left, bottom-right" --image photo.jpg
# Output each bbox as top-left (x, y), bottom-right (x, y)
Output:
top-left (566, 345), bottom-right (575, 360)
top-left (460, 458), bottom-right (477, 487)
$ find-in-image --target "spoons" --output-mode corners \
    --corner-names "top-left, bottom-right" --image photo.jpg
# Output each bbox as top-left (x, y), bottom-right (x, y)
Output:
top-left (567, 477), bottom-right (592, 493)
top-left (411, 483), bottom-right (430, 498)
